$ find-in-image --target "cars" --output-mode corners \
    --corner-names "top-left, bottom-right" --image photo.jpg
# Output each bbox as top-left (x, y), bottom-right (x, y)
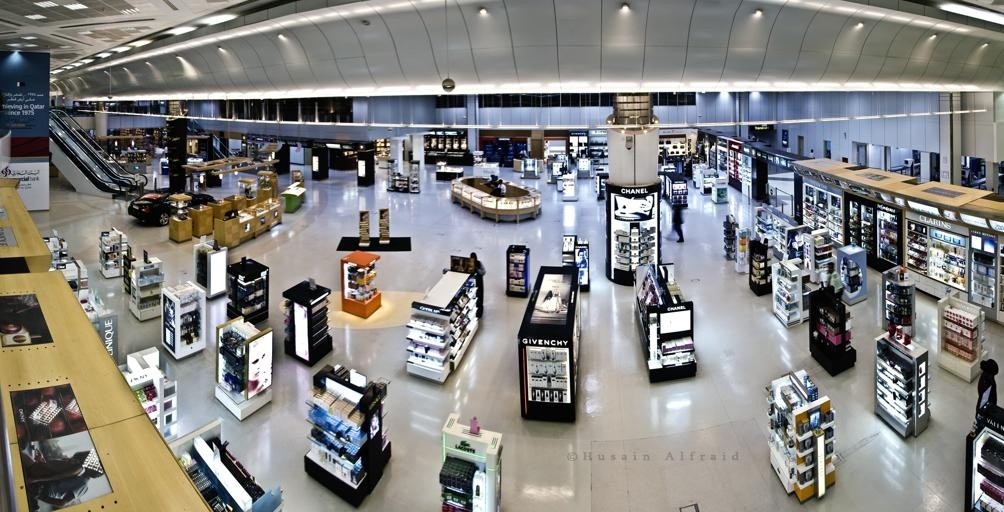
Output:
top-left (128, 188), bottom-right (216, 227)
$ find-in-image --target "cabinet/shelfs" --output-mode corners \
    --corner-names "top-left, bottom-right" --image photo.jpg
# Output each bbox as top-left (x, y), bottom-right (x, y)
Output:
top-left (386, 158), bottom-right (420, 194)
top-left (540, 133), bottom-right (752, 210)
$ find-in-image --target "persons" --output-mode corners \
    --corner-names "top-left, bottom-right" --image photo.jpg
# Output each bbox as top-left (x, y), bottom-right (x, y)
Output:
top-left (519, 146), bottom-right (530, 160)
top-left (479, 173), bottom-right (507, 197)
top-left (671, 198), bottom-right (686, 243)
top-left (974, 358), bottom-right (1000, 418)
top-left (469, 251), bottom-right (488, 316)
